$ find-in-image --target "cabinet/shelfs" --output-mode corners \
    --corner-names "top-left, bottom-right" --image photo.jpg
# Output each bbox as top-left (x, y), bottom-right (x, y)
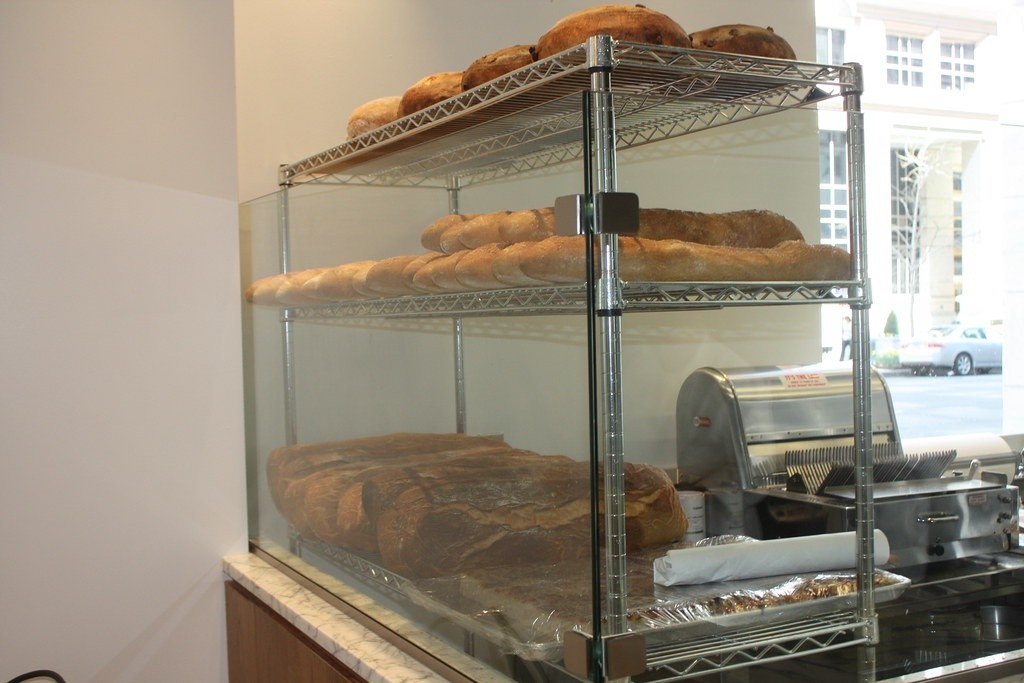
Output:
top-left (240, 34), bottom-right (882, 683)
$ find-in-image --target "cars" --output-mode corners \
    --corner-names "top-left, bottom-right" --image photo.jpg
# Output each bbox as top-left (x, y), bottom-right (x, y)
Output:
top-left (899, 325), bottom-right (1002, 375)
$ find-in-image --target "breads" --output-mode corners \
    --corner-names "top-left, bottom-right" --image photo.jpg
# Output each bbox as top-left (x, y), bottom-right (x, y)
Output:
top-left (265, 433), bottom-right (689, 581)
top-left (346, 4), bottom-right (798, 158)
top-left (244, 208), bottom-right (852, 305)
